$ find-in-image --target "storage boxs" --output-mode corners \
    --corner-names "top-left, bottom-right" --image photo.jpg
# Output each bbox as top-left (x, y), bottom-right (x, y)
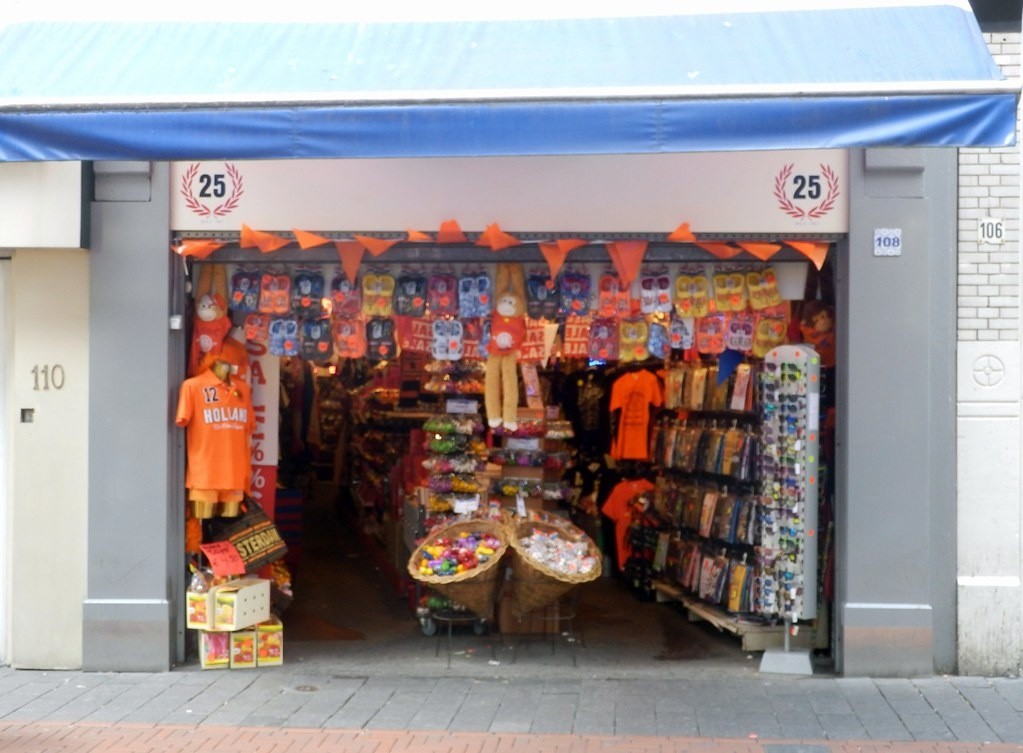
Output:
top-left (186, 579), bottom-right (283, 669)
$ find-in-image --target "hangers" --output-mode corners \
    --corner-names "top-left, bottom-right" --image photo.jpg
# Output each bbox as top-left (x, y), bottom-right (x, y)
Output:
top-left (626, 361), bottom-right (643, 373)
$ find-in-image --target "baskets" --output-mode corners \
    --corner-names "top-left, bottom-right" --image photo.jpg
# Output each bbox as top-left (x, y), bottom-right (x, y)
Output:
top-left (506, 520), bottom-right (602, 623)
top-left (408, 519), bottom-right (509, 621)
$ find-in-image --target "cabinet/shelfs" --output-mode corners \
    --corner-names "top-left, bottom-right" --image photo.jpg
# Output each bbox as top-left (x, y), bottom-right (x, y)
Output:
top-left (328, 352), bottom-right (577, 642)
top-left (608, 338), bottom-right (831, 674)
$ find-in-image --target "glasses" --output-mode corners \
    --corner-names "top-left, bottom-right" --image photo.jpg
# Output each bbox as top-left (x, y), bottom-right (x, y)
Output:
top-left (753, 360), bottom-right (806, 613)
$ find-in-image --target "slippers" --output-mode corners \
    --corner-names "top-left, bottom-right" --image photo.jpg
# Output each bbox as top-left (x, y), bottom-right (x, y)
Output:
top-left (228, 269), bottom-right (790, 362)
